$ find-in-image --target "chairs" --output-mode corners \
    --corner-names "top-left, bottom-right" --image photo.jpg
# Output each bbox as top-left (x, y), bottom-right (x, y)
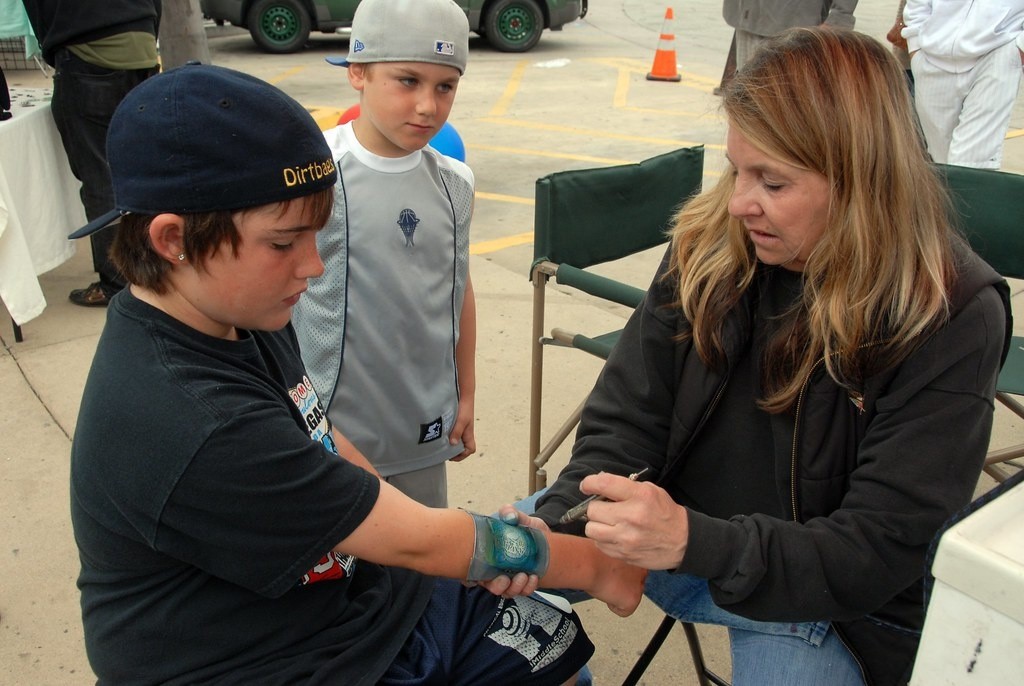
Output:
top-left (528, 146), bottom-right (705, 493)
top-left (931, 162), bottom-right (1024, 484)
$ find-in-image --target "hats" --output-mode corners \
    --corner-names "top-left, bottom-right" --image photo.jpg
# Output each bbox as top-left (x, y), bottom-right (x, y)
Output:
top-left (324, 0), bottom-right (470, 75)
top-left (67, 59), bottom-right (338, 241)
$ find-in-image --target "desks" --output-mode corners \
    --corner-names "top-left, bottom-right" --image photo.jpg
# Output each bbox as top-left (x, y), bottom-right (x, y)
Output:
top-left (0, 86), bottom-right (86, 343)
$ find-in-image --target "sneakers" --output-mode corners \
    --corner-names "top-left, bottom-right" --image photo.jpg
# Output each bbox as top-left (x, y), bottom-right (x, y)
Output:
top-left (69, 280), bottom-right (110, 305)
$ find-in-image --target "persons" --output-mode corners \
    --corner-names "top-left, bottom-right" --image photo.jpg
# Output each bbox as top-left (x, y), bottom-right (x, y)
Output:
top-left (722, 0), bottom-right (859, 70)
top-left (23, 0), bottom-right (162, 307)
top-left (462, 26), bottom-right (1014, 686)
top-left (900, 1), bottom-right (1024, 171)
top-left (283, 0), bottom-right (476, 508)
top-left (68, 63), bottom-right (647, 686)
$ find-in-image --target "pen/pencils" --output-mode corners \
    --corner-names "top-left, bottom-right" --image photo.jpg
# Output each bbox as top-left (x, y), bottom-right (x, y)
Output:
top-left (558, 465), bottom-right (653, 526)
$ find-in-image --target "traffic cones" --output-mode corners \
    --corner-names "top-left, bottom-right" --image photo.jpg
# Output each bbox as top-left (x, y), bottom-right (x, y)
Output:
top-left (646, 6), bottom-right (681, 85)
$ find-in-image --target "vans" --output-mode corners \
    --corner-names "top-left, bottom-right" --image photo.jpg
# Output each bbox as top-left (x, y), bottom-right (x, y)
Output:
top-left (198, 1), bottom-right (589, 53)
